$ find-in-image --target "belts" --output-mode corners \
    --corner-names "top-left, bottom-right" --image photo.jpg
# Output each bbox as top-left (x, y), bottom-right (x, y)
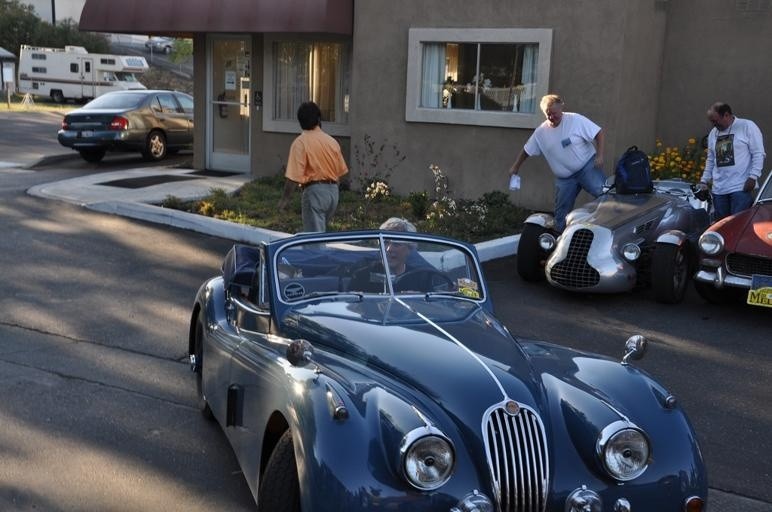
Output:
top-left (298, 179), bottom-right (339, 188)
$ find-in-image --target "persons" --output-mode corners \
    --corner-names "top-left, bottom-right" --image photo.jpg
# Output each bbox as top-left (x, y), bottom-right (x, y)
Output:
top-left (277, 102), bottom-right (348, 232)
top-left (348, 216), bottom-right (439, 293)
top-left (697, 104), bottom-right (766, 220)
top-left (509, 93), bottom-right (608, 228)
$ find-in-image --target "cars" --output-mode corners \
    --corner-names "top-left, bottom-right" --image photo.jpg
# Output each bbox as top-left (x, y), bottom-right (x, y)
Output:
top-left (57, 89), bottom-right (194, 163)
top-left (144, 37), bottom-right (193, 55)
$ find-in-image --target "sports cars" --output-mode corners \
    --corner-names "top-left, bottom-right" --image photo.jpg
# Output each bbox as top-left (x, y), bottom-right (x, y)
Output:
top-left (695, 167), bottom-right (771, 308)
top-left (187, 231), bottom-right (710, 510)
top-left (517, 174), bottom-right (716, 307)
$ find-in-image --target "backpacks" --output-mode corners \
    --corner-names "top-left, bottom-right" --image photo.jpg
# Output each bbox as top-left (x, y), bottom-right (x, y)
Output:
top-left (608, 145), bottom-right (656, 196)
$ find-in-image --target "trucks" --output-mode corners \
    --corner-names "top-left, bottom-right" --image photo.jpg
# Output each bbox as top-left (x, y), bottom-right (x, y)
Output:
top-left (18, 43), bottom-right (149, 104)
